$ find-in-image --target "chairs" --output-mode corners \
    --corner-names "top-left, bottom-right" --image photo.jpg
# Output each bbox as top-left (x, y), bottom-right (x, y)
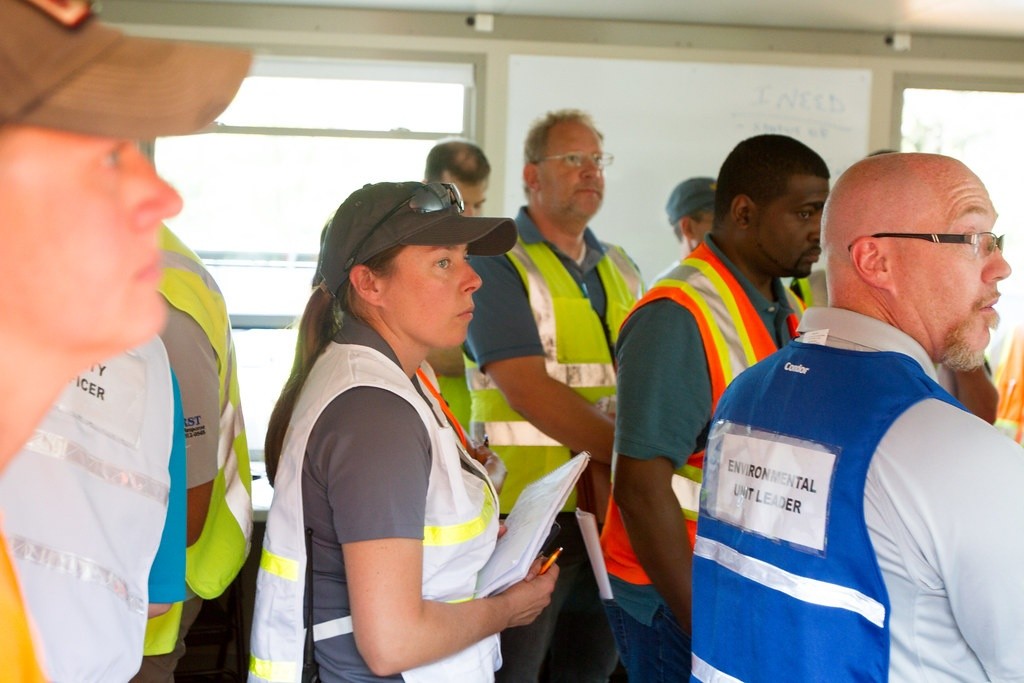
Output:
top-left (171, 573), bottom-right (245, 682)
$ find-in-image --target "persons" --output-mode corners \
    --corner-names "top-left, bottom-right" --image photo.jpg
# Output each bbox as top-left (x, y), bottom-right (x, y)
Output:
top-left (995, 325), bottom-right (1024, 445)
top-left (459, 109), bottom-right (649, 683)
top-left (248, 181), bottom-right (560, 683)
top-left (649, 175), bottom-right (716, 287)
top-left (425, 141), bottom-right (490, 433)
top-left (131, 218), bottom-right (252, 682)
top-left (693, 154), bottom-right (1024, 683)
top-left (416, 356), bottom-right (508, 495)
top-left (603, 134), bottom-right (830, 682)
top-left (0, 2), bottom-right (256, 683)
top-left (0, 333), bottom-right (188, 683)
top-left (933, 352), bottom-right (998, 425)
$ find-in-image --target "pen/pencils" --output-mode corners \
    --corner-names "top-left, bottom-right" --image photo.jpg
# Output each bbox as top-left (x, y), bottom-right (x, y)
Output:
top-left (484, 435), bottom-right (489, 447)
top-left (537, 546), bottom-right (564, 575)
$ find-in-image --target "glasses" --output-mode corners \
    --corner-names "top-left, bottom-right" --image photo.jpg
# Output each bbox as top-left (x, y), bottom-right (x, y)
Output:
top-left (544, 151), bottom-right (614, 167)
top-left (342, 181), bottom-right (464, 271)
top-left (848, 231), bottom-right (1004, 259)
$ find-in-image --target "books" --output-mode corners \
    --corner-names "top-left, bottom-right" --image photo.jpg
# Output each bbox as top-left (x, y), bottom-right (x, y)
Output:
top-left (474, 451), bottom-right (590, 597)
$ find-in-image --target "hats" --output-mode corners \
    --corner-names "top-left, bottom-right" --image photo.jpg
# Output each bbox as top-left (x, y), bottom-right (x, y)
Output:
top-left (320, 182), bottom-right (518, 301)
top-left (666, 177), bottom-right (716, 225)
top-left (0, 0), bottom-right (252, 139)
